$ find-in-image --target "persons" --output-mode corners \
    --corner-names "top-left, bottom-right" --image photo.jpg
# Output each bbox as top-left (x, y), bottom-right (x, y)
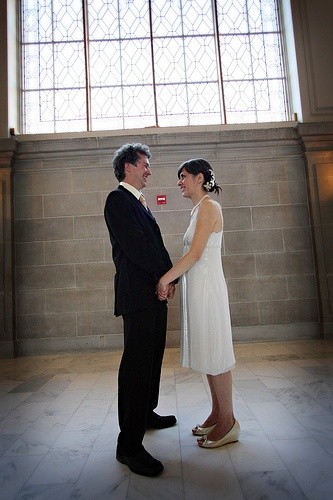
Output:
top-left (102, 142), bottom-right (181, 478)
top-left (158, 159), bottom-right (241, 450)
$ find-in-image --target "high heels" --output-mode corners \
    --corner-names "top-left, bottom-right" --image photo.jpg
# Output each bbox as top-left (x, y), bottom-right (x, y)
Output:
top-left (197, 420), bottom-right (239, 448)
top-left (191, 423), bottom-right (216, 436)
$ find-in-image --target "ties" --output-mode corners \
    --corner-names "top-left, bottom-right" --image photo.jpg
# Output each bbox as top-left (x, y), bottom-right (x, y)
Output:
top-left (138, 195), bottom-right (147, 211)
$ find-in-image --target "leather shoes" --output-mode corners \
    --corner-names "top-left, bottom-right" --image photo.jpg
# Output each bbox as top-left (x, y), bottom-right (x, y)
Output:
top-left (115, 447), bottom-right (164, 477)
top-left (144, 411), bottom-right (176, 429)
